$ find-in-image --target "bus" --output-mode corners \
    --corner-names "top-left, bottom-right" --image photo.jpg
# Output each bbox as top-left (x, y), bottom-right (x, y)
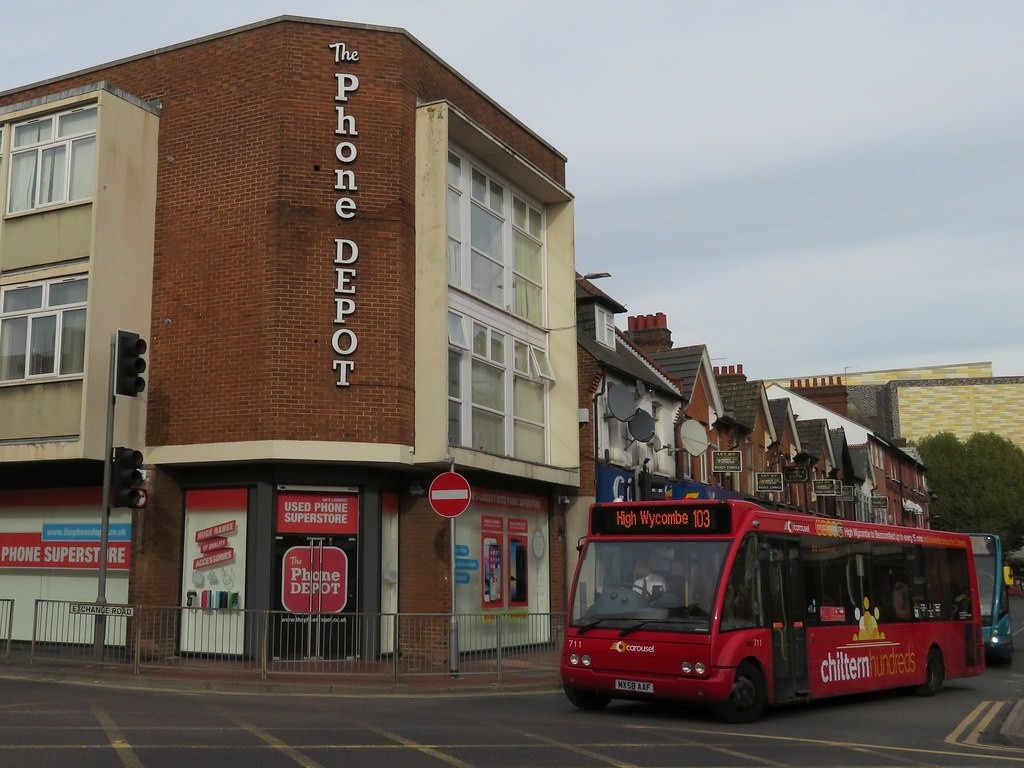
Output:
top-left (560, 499), bottom-right (986, 723)
top-left (961, 533), bottom-right (1015, 666)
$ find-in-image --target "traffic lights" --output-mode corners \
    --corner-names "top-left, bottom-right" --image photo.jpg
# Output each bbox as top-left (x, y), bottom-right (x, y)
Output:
top-left (116, 331), bottom-right (146, 398)
top-left (111, 447), bottom-right (144, 510)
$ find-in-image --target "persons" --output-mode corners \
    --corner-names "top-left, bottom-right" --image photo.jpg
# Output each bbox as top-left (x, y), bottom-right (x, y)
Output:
top-left (632, 559), bottom-right (666, 599)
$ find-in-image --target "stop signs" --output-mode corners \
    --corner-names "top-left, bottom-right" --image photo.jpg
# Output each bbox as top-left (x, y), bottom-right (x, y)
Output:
top-left (428, 472), bottom-right (472, 517)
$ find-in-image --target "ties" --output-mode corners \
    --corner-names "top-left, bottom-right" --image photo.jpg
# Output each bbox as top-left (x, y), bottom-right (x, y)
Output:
top-left (642, 579), bottom-right (646, 598)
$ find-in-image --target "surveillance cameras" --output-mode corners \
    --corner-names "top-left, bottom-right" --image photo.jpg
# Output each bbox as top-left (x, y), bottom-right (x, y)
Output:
top-left (563, 498), bottom-right (570, 505)
